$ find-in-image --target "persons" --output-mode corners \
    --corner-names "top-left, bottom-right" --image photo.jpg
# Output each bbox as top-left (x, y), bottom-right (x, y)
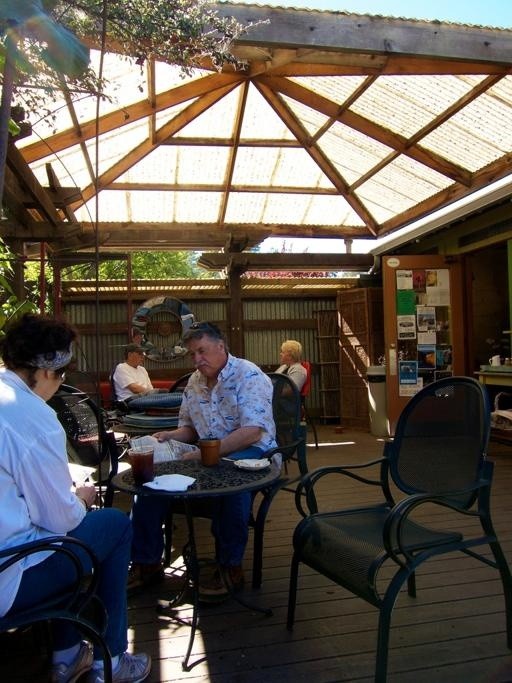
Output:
top-left (273, 340), bottom-right (307, 416)
top-left (2, 307), bottom-right (154, 683)
top-left (124, 321), bottom-right (283, 596)
top-left (110, 344), bottom-right (155, 409)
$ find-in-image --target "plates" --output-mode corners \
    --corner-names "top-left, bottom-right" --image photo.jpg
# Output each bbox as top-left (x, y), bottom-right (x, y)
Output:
top-left (235, 458), bottom-right (270, 470)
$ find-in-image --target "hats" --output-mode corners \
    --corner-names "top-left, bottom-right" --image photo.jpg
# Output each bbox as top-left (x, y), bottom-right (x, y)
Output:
top-left (125, 343), bottom-right (148, 353)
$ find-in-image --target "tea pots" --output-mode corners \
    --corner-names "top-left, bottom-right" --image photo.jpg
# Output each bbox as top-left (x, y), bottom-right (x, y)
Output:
top-left (489, 355), bottom-right (501, 367)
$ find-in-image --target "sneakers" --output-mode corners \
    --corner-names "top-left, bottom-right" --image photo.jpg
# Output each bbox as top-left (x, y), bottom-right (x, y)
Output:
top-left (126, 562), bottom-right (166, 589)
top-left (199, 568), bottom-right (247, 596)
top-left (91, 652), bottom-right (152, 683)
top-left (53, 640), bottom-right (93, 683)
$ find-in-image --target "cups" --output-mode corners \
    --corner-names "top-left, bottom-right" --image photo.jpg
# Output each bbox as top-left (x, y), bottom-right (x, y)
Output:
top-left (127, 445), bottom-right (154, 484)
top-left (200, 438), bottom-right (221, 466)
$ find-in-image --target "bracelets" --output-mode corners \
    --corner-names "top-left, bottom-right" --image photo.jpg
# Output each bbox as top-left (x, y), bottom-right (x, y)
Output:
top-left (81, 498), bottom-right (89, 508)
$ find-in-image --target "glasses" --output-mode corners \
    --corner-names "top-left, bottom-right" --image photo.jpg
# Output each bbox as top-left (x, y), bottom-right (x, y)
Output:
top-left (189, 321), bottom-right (218, 336)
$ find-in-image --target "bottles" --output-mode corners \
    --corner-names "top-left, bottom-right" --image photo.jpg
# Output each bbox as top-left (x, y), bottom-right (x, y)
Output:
top-left (504, 357), bottom-right (509, 365)
